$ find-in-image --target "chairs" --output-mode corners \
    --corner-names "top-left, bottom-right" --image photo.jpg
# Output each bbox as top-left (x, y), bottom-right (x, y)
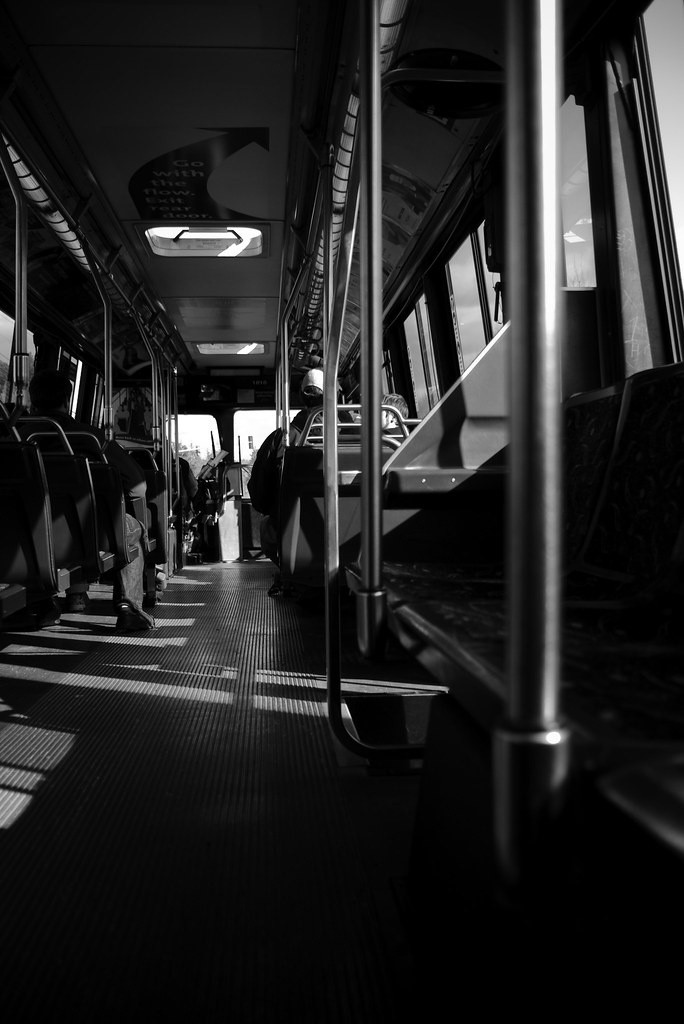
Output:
top-left (273, 404), bottom-right (410, 590)
top-left (0, 401), bottom-right (189, 620)
top-left (345, 362), bottom-right (684, 862)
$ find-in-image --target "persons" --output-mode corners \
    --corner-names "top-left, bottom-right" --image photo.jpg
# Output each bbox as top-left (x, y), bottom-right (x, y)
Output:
top-left (26, 367), bottom-right (205, 631)
top-left (247, 368), bottom-right (413, 597)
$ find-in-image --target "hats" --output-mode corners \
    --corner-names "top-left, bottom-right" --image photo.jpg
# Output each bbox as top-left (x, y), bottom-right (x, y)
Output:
top-left (381, 393), bottom-right (409, 422)
top-left (301, 367), bottom-right (342, 395)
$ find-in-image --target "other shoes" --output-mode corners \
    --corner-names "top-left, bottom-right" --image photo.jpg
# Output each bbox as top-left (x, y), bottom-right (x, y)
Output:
top-left (116, 604), bottom-right (151, 631)
top-left (65, 592), bottom-right (95, 612)
top-left (38, 616), bottom-right (62, 627)
top-left (142, 590), bottom-right (165, 601)
